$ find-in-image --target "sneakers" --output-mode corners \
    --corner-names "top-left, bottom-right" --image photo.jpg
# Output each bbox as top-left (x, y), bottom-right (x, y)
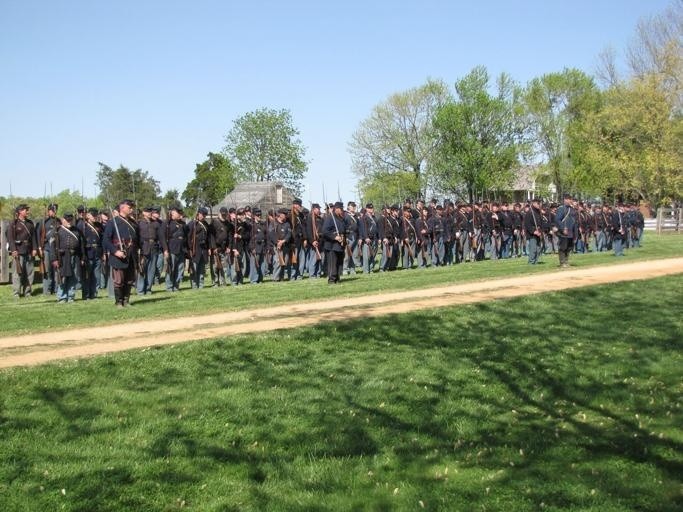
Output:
top-left (560, 262), bottom-right (570, 267)
top-left (58, 299), bottom-right (77, 305)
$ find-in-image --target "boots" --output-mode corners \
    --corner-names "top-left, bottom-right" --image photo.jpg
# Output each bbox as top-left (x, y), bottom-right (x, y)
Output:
top-left (114, 285), bottom-right (135, 310)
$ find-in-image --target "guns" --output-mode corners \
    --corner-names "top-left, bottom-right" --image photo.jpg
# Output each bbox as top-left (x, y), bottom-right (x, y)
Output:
top-left (9, 174), bottom-right (641, 286)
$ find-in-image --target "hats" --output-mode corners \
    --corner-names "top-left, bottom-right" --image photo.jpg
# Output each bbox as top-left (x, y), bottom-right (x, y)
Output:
top-left (48, 203), bottom-right (58, 210)
top-left (112, 205), bottom-right (120, 213)
top-left (153, 205), bottom-right (161, 213)
top-left (63, 212), bottom-right (73, 221)
top-left (142, 206), bottom-right (153, 212)
top-left (15, 204), bottom-right (30, 212)
top-left (78, 205), bottom-right (110, 214)
top-left (381, 199), bottom-right (530, 212)
top-left (531, 194), bottom-right (640, 208)
top-left (119, 199), bottom-right (136, 207)
top-left (220, 206), bottom-right (262, 216)
top-left (268, 199), bottom-right (374, 217)
top-left (198, 207), bottom-right (208, 215)
top-left (169, 205), bottom-right (184, 214)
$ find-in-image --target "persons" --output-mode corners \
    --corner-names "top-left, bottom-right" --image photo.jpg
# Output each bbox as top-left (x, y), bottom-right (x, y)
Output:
top-left (4, 193), bottom-right (646, 311)
